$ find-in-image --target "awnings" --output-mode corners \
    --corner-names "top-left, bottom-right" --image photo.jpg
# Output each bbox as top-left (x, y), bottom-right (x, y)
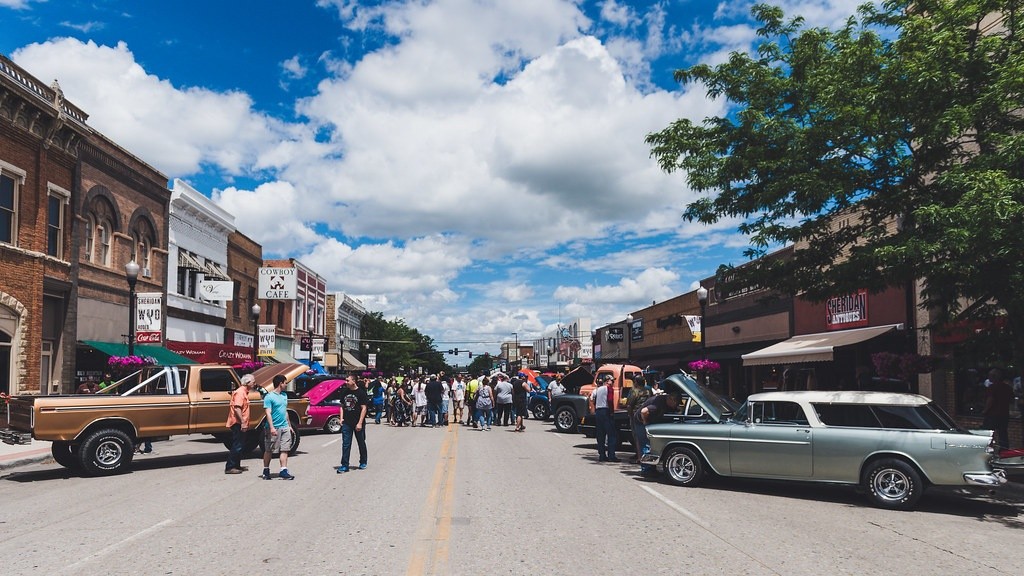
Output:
top-left (741, 323), bottom-right (906, 366)
top-left (258, 349), bottom-right (303, 366)
top-left (77, 340), bottom-right (201, 381)
top-left (296, 360), bottom-right (333, 379)
top-left (166, 340), bottom-right (264, 377)
top-left (339, 352), bottom-right (366, 371)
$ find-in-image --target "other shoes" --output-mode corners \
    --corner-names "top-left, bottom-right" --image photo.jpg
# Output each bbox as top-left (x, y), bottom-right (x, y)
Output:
top-left (599, 456), bottom-right (608, 462)
top-left (225, 466), bottom-right (248, 473)
top-left (375, 417), bottom-right (526, 432)
top-left (608, 456), bottom-right (622, 462)
top-left (630, 456), bottom-right (640, 464)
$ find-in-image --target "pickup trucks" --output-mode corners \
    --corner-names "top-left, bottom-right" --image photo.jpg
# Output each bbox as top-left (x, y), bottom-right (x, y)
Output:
top-left (1, 361), bottom-right (312, 476)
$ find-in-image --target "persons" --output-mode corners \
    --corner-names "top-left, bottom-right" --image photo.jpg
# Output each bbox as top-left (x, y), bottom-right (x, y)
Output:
top-left (626, 375), bottom-right (647, 464)
top-left (373, 371), bottom-right (538, 428)
top-left (337, 374), bottom-right (367, 473)
top-left (513, 374), bottom-right (530, 432)
top-left (978, 368), bottom-right (1013, 454)
top-left (262, 375), bottom-right (294, 480)
top-left (474, 377), bottom-right (495, 431)
top-left (594, 375), bottom-right (622, 462)
top-left (78, 370), bottom-right (160, 456)
top-left (225, 374), bottom-right (256, 475)
top-left (650, 383), bottom-right (660, 396)
top-left (548, 374), bottom-right (569, 406)
top-left (634, 392), bottom-right (677, 464)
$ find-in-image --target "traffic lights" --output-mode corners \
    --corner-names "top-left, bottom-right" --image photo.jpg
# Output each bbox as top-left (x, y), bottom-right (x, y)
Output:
top-left (455, 349), bottom-right (458, 355)
top-left (469, 353), bottom-right (472, 358)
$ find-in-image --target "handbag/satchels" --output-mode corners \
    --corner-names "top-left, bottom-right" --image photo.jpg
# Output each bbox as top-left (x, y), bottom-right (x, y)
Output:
top-left (371, 397), bottom-right (383, 406)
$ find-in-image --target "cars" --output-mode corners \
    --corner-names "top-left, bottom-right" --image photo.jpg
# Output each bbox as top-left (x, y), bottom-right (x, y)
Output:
top-left (295, 380), bottom-right (346, 433)
top-left (521, 362), bottom-right (769, 448)
top-left (640, 371), bottom-right (1006, 507)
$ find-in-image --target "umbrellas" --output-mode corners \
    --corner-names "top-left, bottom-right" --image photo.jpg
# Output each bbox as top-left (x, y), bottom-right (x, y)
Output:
top-left (942, 350), bottom-right (981, 372)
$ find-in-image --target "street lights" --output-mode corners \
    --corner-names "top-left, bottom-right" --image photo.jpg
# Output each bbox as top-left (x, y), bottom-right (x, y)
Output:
top-left (547, 347), bottom-right (551, 367)
top-left (697, 287), bottom-right (708, 360)
top-left (308, 325), bottom-right (314, 362)
top-left (377, 348), bottom-right (381, 371)
top-left (365, 344), bottom-right (369, 371)
top-left (512, 333), bottom-right (518, 375)
top-left (626, 312), bottom-right (634, 366)
top-left (535, 350), bottom-right (539, 367)
top-left (125, 261), bottom-right (140, 355)
top-left (340, 336), bottom-right (344, 370)
top-left (252, 304), bottom-right (261, 361)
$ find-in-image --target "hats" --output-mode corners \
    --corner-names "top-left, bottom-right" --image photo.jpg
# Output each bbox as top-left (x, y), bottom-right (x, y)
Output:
top-left (519, 372), bottom-right (528, 378)
top-left (602, 375), bottom-right (617, 382)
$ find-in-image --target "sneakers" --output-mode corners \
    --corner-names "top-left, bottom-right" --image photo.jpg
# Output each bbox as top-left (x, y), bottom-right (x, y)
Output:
top-left (278, 470), bottom-right (294, 480)
top-left (263, 468), bottom-right (272, 480)
top-left (359, 462), bottom-right (368, 469)
top-left (337, 464), bottom-right (350, 473)
